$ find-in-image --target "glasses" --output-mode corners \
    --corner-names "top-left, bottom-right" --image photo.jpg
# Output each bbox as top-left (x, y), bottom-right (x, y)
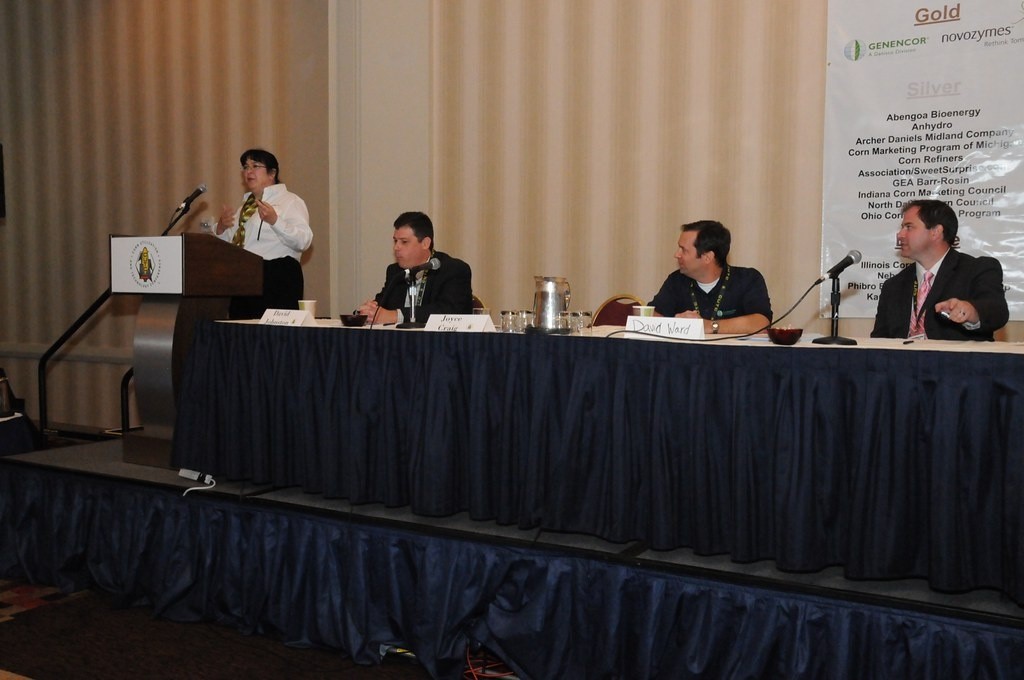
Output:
top-left (240, 165), bottom-right (266, 171)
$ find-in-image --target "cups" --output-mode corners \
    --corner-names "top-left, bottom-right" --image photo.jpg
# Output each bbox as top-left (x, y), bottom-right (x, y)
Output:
top-left (559, 312), bottom-right (593, 335)
top-left (298, 300), bottom-right (316, 320)
top-left (500, 310), bottom-right (534, 333)
top-left (639, 306), bottom-right (655, 317)
top-left (473, 308), bottom-right (490, 314)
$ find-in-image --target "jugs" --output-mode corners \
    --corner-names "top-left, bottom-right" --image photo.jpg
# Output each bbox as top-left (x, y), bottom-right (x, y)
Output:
top-left (533, 275), bottom-right (571, 334)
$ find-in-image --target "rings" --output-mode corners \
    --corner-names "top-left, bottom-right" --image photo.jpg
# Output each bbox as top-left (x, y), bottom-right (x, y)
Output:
top-left (959, 311), bottom-right (965, 316)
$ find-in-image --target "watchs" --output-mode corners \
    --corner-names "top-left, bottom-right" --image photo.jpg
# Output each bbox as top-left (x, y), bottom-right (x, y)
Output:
top-left (710, 320), bottom-right (719, 334)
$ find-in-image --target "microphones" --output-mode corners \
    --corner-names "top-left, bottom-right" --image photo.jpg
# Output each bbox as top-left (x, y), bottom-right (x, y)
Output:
top-left (815, 250), bottom-right (862, 286)
top-left (401, 258), bottom-right (441, 275)
top-left (176, 184), bottom-right (207, 212)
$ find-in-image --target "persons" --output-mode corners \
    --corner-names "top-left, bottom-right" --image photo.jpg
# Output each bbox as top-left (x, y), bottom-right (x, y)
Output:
top-left (360, 212), bottom-right (473, 326)
top-left (647, 221), bottom-right (773, 335)
top-left (869, 199), bottom-right (1009, 342)
top-left (209, 149), bottom-right (313, 319)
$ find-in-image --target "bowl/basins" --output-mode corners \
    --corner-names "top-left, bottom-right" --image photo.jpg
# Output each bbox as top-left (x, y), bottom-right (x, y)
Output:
top-left (340, 315), bottom-right (368, 326)
top-left (767, 328), bottom-right (803, 345)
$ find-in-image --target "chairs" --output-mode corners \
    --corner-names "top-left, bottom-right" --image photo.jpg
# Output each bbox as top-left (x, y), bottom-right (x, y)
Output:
top-left (592, 295), bottom-right (643, 329)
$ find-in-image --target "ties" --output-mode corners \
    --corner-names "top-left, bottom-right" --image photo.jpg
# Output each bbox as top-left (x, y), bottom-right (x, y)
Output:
top-left (232, 194), bottom-right (262, 249)
top-left (909, 272), bottom-right (934, 339)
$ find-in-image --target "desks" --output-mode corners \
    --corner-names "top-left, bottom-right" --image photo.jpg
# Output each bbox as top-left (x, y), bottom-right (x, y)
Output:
top-left (173, 311), bottom-right (1024, 607)
